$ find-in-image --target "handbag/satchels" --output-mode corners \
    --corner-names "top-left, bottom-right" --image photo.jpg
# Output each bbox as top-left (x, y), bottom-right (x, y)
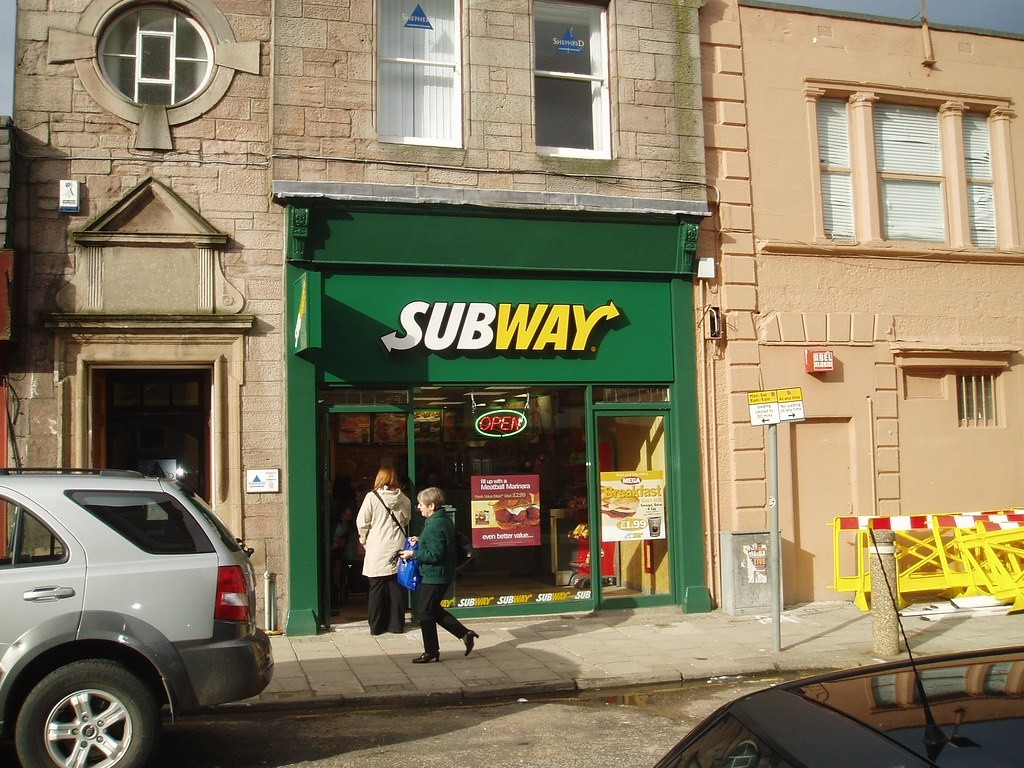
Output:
top-left (396, 539), bottom-right (420, 591)
top-left (451, 518), bottom-right (479, 569)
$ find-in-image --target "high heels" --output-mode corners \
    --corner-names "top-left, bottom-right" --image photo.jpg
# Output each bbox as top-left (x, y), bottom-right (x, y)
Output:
top-left (413, 650), bottom-right (440, 663)
top-left (464, 630), bottom-right (479, 656)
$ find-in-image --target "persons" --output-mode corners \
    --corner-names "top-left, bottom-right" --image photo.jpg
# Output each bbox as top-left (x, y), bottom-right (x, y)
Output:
top-left (320, 459), bottom-right (362, 616)
top-left (397, 488), bottom-right (480, 663)
top-left (354, 466), bottom-right (413, 634)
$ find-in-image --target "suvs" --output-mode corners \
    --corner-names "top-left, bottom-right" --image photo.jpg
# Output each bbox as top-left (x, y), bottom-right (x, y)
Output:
top-left (0, 466), bottom-right (275, 768)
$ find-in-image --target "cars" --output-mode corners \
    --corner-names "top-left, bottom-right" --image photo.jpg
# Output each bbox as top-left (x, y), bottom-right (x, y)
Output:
top-left (656, 642), bottom-right (1024, 768)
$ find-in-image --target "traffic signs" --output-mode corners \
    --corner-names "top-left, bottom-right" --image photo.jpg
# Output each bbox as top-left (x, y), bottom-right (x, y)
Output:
top-left (779, 401), bottom-right (805, 421)
top-left (750, 402), bottom-right (781, 427)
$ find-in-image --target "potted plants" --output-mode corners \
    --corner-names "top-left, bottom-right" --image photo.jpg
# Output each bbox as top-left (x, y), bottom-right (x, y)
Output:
top-left (567, 496), bottom-right (588, 524)
